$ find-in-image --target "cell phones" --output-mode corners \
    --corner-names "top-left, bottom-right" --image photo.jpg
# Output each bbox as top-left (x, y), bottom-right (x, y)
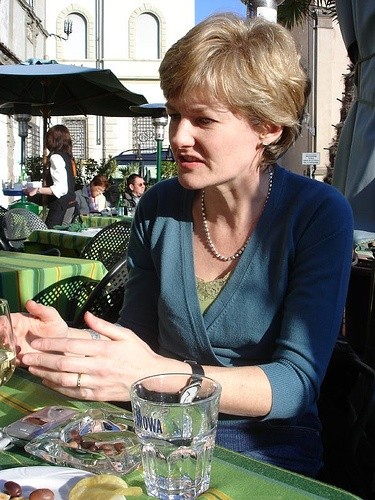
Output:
top-left (2, 406), bottom-right (76, 442)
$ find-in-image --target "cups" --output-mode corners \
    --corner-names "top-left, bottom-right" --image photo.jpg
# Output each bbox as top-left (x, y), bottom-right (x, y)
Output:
top-left (2, 179), bottom-right (12, 189)
top-left (131, 372), bottom-right (223, 500)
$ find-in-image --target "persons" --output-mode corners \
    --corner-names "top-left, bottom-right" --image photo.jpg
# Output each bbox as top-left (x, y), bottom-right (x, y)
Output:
top-left (124, 174), bottom-right (146, 215)
top-left (73, 175), bottom-right (110, 216)
top-left (0, 12), bottom-right (354, 481)
top-left (25, 124), bottom-right (78, 231)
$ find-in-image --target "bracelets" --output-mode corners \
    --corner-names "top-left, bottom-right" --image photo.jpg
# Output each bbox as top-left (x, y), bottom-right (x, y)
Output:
top-left (83, 329), bottom-right (101, 341)
top-left (36, 188), bottom-right (40, 194)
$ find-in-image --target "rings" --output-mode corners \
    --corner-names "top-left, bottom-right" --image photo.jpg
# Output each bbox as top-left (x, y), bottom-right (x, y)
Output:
top-left (77, 372), bottom-right (81, 389)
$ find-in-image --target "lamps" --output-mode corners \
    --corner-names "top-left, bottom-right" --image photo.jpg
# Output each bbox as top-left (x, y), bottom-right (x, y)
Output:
top-left (49, 17), bottom-right (73, 41)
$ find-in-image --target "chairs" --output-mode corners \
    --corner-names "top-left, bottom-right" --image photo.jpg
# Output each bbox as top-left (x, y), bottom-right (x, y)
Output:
top-left (33, 220), bottom-right (131, 328)
top-left (318, 334), bottom-right (375, 497)
top-left (1, 207), bottom-right (131, 328)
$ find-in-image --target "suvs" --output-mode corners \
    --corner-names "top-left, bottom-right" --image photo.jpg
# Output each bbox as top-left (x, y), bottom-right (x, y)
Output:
top-left (107, 145), bottom-right (175, 186)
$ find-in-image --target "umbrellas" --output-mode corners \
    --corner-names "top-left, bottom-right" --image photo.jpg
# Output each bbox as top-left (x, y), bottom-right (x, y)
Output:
top-left (329, 0), bottom-right (375, 234)
top-left (0, 58), bottom-right (147, 227)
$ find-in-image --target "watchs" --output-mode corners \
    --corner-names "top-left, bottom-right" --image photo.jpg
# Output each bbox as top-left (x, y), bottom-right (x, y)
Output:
top-left (178, 359), bottom-right (204, 405)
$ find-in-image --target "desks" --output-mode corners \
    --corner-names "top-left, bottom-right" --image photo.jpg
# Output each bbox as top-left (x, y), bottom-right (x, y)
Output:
top-left (1, 369), bottom-right (362, 500)
top-left (0, 249), bottom-right (109, 312)
top-left (82, 213), bottom-right (133, 228)
top-left (30, 225), bottom-right (105, 258)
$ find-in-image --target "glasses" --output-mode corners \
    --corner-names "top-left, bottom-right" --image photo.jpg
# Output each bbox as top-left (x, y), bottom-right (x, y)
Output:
top-left (135, 182), bottom-right (146, 187)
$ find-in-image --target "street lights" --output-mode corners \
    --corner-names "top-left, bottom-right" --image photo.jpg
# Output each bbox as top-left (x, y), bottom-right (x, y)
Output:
top-left (152, 117), bottom-right (168, 182)
top-left (8, 114), bottom-right (43, 216)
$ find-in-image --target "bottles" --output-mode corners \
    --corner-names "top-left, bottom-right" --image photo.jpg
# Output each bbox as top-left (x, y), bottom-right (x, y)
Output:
top-left (117, 193), bottom-right (125, 217)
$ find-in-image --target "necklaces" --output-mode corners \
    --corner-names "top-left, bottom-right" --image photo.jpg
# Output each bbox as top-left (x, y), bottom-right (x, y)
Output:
top-left (201, 167), bottom-right (273, 261)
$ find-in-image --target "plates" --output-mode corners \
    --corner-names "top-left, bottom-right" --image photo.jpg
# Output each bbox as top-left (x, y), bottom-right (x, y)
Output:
top-left (0, 467), bottom-right (128, 500)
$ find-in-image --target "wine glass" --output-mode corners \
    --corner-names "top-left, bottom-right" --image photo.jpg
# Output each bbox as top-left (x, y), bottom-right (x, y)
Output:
top-left (0, 298), bottom-right (19, 450)
